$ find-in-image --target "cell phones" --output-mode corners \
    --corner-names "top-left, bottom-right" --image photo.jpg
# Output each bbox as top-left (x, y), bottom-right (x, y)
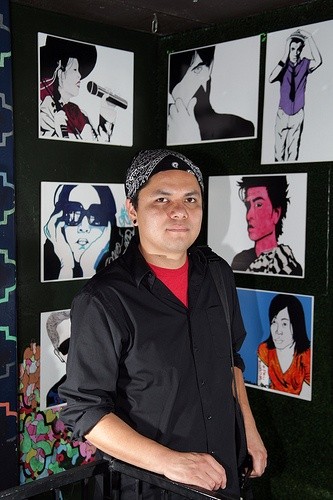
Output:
top-left (238, 455), bottom-right (253, 489)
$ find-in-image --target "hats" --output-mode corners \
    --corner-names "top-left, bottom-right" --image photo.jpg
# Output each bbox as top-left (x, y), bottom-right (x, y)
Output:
top-left (126, 150), bottom-right (205, 198)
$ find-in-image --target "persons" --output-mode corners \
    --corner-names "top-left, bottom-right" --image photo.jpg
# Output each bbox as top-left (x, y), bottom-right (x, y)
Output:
top-left (60, 150), bottom-right (270, 500)
